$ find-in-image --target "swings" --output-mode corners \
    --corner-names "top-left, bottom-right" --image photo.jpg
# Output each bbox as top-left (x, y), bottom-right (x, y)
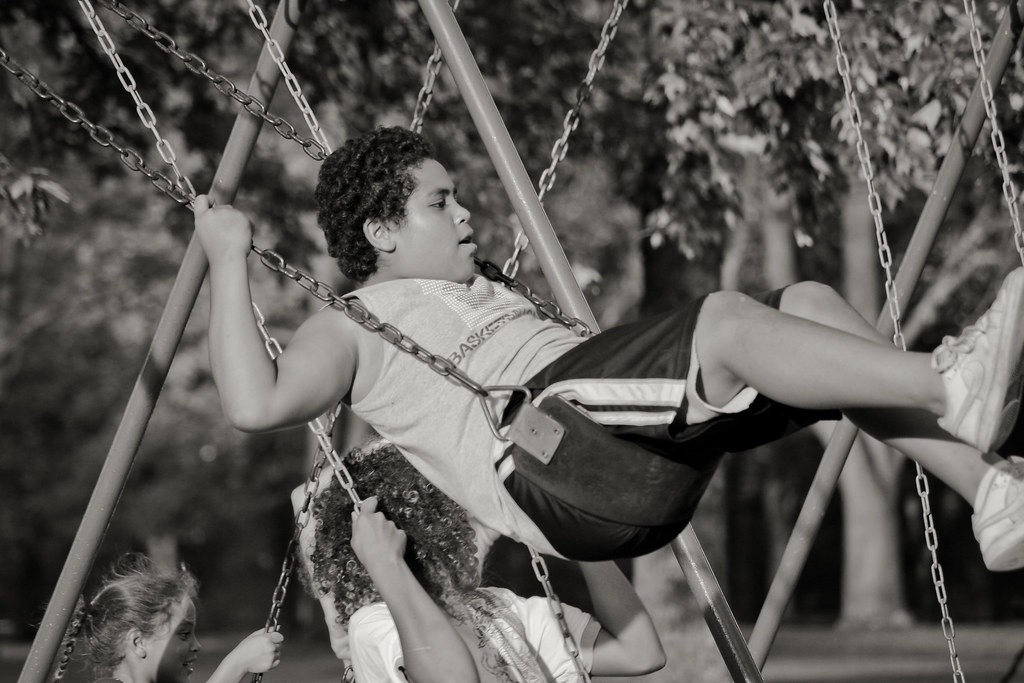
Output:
top-left (1, 0), bottom-right (1023, 683)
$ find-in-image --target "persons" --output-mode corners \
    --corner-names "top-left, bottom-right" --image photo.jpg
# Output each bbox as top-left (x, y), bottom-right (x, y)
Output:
top-left (307, 443), bottom-right (667, 683)
top-left (49, 570), bottom-right (284, 683)
top-left (192, 127), bottom-right (1023, 573)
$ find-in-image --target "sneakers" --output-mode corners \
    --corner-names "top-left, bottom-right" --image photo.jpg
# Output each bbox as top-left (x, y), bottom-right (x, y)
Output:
top-left (931, 261), bottom-right (1024, 454)
top-left (971, 455), bottom-right (1023, 571)
